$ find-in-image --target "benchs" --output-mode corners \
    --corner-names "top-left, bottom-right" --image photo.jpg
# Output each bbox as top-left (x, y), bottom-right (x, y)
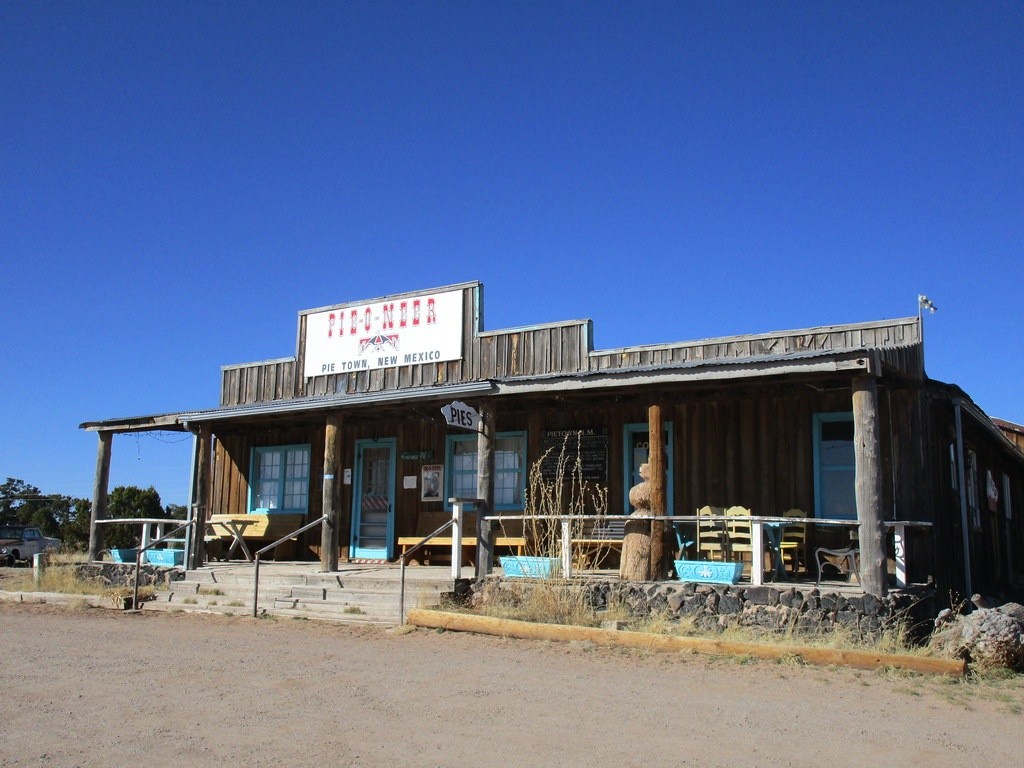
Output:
top-left (398, 536), bottom-right (526, 557)
top-left (207, 513), bottom-right (306, 561)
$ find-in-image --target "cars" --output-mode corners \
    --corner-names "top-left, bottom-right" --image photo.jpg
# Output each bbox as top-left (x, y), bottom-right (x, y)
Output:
top-left (0, 525), bottom-right (61, 568)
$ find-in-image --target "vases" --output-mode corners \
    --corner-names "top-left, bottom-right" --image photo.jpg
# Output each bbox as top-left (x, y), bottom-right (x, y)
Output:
top-left (499, 555), bottom-right (560, 579)
top-left (673, 559), bottom-right (744, 585)
top-left (145, 547), bottom-right (185, 566)
top-left (110, 549), bottom-right (139, 563)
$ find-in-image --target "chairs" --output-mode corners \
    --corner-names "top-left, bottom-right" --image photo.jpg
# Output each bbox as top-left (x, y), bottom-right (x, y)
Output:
top-left (724, 505), bottom-right (773, 584)
top-left (697, 505), bottom-right (726, 563)
top-left (769, 508), bottom-right (808, 583)
top-left (814, 520), bottom-right (884, 586)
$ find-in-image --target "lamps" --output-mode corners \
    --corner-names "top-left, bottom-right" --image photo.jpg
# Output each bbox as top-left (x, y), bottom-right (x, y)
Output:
top-left (918, 293), bottom-right (938, 343)
